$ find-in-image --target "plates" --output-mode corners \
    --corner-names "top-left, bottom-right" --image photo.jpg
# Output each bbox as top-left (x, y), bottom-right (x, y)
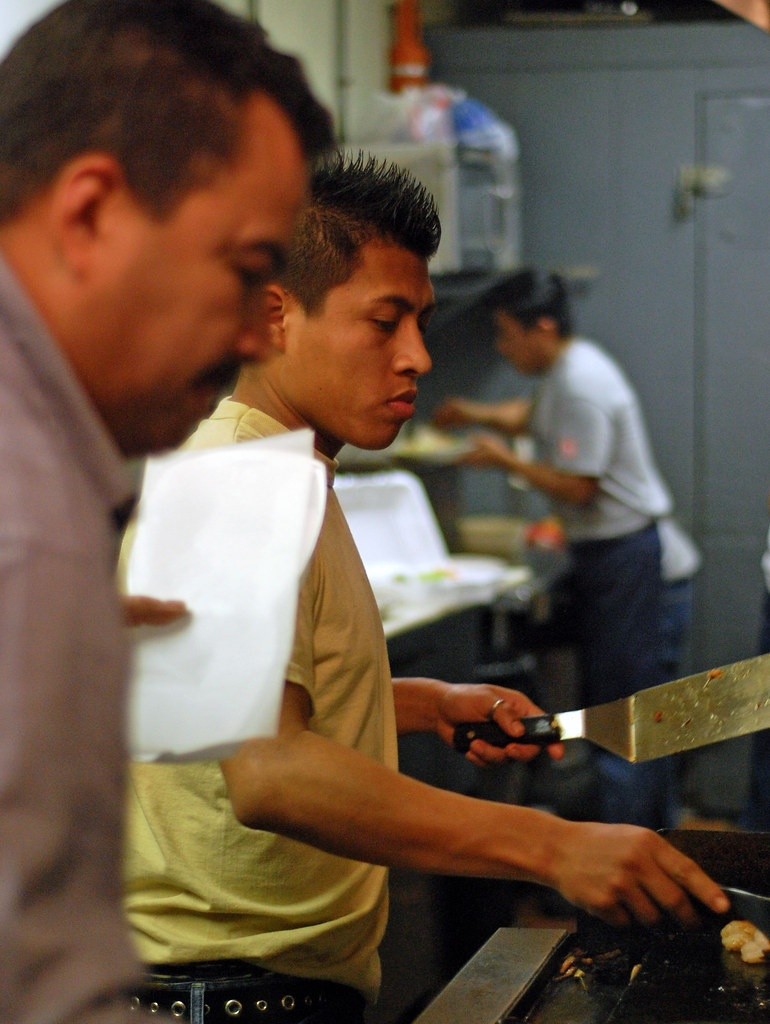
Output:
top-left (397, 437), bottom-right (492, 467)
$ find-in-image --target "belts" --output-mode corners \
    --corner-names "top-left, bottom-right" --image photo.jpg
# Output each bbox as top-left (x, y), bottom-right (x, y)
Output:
top-left (129, 983), bottom-right (338, 1024)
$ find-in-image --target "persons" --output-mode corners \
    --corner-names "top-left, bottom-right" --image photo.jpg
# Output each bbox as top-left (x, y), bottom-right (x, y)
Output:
top-left (116, 151), bottom-right (730, 1024)
top-left (0, 0), bottom-right (335, 1024)
top-left (431, 273), bottom-right (704, 830)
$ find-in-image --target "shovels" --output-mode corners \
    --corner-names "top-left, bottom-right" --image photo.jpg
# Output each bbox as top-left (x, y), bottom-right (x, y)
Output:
top-left (454, 651), bottom-right (770, 766)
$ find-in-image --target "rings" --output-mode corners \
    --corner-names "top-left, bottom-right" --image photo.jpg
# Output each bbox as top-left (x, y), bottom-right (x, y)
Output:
top-left (487, 698), bottom-right (505, 719)
